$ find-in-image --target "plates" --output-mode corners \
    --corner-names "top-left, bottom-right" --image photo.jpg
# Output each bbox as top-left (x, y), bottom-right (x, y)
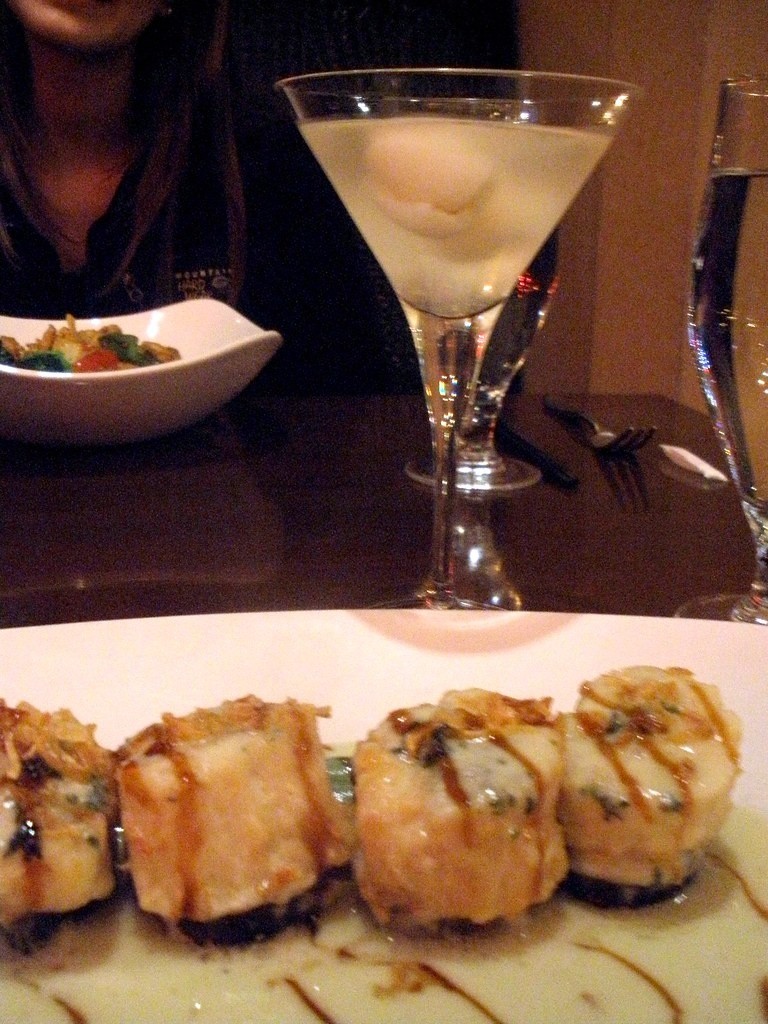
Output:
top-left (0, 609), bottom-right (768, 1024)
top-left (0, 297), bottom-right (283, 447)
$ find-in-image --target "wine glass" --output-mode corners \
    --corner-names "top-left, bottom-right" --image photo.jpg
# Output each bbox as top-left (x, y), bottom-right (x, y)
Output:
top-left (674, 75), bottom-right (768, 627)
top-left (273, 66), bottom-right (643, 610)
top-left (402, 102), bottom-right (562, 491)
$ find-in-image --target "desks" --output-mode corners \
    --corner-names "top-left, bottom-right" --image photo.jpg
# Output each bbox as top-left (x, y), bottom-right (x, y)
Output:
top-left (0, 395), bottom-right (768, 1024)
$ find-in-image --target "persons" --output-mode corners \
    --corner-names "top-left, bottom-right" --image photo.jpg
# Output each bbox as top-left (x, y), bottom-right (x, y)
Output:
top-left (1, 2), bottom-right (429, 398)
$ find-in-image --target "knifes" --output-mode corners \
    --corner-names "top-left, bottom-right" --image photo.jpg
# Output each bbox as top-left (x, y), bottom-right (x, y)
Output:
top-left (495, 424), bottom-right (578, 491)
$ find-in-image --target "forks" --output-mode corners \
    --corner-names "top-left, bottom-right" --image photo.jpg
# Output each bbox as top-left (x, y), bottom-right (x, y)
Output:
top-left (543, 392), bottom-right (658, 457)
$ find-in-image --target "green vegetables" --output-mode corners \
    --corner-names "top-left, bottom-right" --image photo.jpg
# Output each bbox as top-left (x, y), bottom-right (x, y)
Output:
top-left (0, 340), bottom-right (69, 372)
top-left (98, 332), bottom-right (157, 368)
